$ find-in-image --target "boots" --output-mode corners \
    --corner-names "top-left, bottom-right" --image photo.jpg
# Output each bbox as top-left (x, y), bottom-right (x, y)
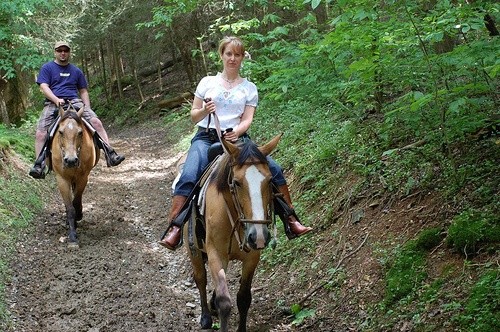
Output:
top-left (276, 183), bottom-right (313, 236)
top-left (163, 195), bottom-right (188, 246)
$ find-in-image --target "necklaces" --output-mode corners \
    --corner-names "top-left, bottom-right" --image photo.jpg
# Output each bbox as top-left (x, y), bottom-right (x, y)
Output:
top-left (221, 76), bottom-right (235, 89)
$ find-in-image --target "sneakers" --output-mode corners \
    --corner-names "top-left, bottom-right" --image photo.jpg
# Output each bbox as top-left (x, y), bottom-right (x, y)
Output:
top-left (109, 152), bottom-right (125, 166)
top-left (29, 163), bottom-right (45, 179)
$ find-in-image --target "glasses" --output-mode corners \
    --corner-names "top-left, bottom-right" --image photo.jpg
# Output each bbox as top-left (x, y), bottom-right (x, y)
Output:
top-left (56, 49), bottom-right (70, 52)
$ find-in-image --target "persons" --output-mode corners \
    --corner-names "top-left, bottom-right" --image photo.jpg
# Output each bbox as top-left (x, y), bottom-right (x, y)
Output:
top-left (164, 38), bottom-right (314, 248)
top-left (29, 40), bottom-right (126, 180)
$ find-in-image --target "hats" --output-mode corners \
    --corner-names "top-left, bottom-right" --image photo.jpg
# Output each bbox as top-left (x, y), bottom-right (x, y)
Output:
top-left (54, 41), bottom-right (71, 50)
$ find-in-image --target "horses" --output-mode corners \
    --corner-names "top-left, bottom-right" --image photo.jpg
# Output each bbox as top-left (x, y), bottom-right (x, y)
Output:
top-left (182, 132), bottom-right (284, 332)
top-left (46, 102), bottom-right (101, 251)
